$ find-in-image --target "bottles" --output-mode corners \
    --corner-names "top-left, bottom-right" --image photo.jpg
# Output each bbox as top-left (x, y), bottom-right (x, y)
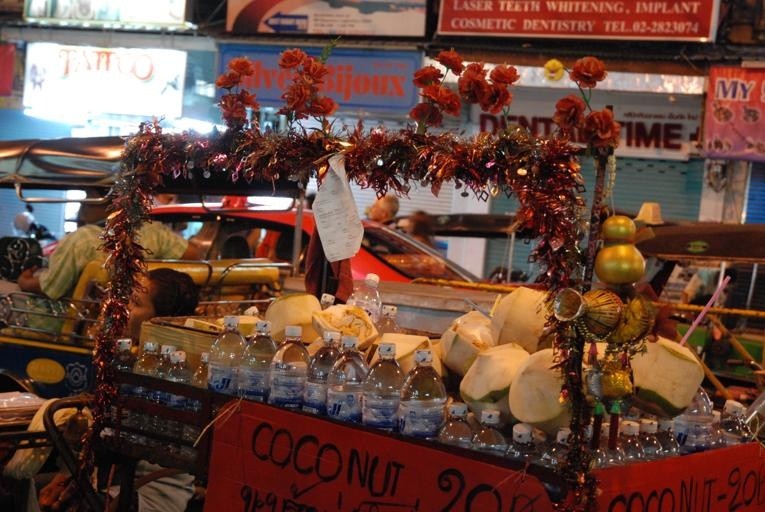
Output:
top-left (438, 390), bottom-right (751, 470)
top-left (111, 337), bottom-right (211, 464)
top-left (319, 273), bottom-right (403, 337)
top-left (207, 313), bottom-right (449, 444)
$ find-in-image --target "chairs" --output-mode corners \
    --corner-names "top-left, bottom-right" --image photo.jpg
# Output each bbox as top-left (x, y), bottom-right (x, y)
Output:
top-left (93, 375), bottom-right (210, 509)
top-left (222, 230), bottom-right (307, 263)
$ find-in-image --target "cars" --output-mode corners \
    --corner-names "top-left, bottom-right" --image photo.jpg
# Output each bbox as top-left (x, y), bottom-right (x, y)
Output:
top-left (39, 194), bottom-right (569, 322)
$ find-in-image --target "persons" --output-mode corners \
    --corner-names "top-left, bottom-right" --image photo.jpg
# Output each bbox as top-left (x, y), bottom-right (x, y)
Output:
top-left (0, 218), bottom-right (202, 334)
top-left (69, 268), bottom-right (199, 512)
top-left (406, 210), bottom-right (441, 250)
top-left (368, 194), bottom-right (401, 230)
